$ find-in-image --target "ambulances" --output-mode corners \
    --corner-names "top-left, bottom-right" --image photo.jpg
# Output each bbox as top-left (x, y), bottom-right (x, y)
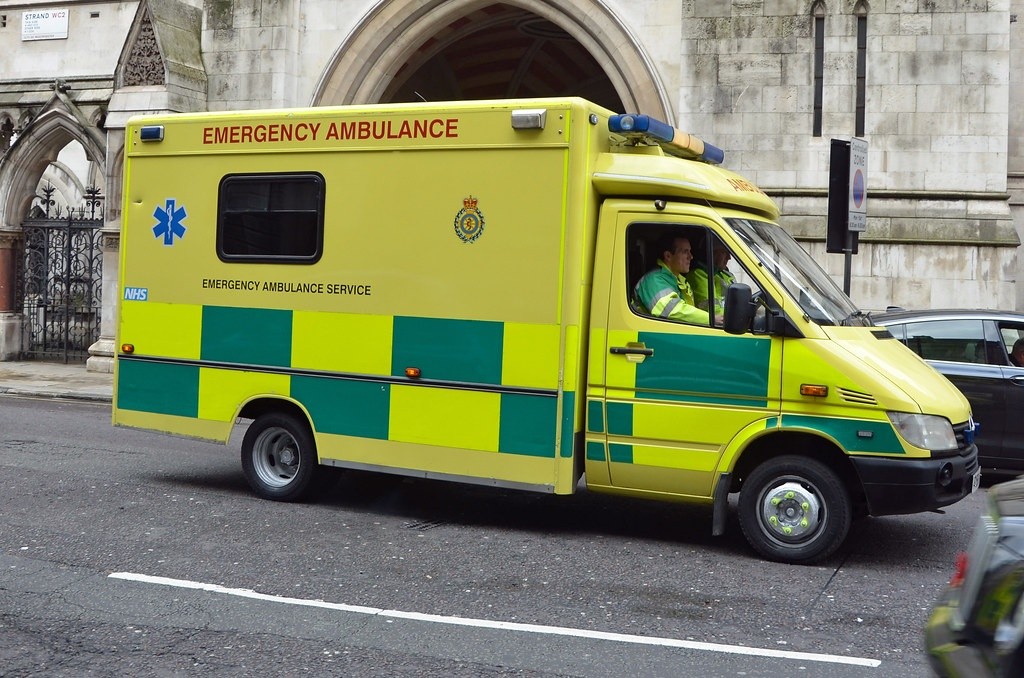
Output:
top-left (111, 97), bottom-right (983, 564)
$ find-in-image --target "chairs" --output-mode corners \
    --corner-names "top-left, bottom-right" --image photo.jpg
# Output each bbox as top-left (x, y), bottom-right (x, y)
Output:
top-left (945, 339), bottom-right (986, 364)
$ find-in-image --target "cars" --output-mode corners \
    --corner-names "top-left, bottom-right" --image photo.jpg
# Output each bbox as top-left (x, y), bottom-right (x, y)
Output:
top-left (921, 478), bottom-right (1024, 678)
top-left (869, 306), bottom-right (1024, 479)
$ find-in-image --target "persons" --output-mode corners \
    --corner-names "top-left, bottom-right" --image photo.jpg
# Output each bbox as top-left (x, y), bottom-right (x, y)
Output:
top-left (1008, 337), bottom-right (1024, 366)
top-left (630, 230), bottom-right (766, 329)
